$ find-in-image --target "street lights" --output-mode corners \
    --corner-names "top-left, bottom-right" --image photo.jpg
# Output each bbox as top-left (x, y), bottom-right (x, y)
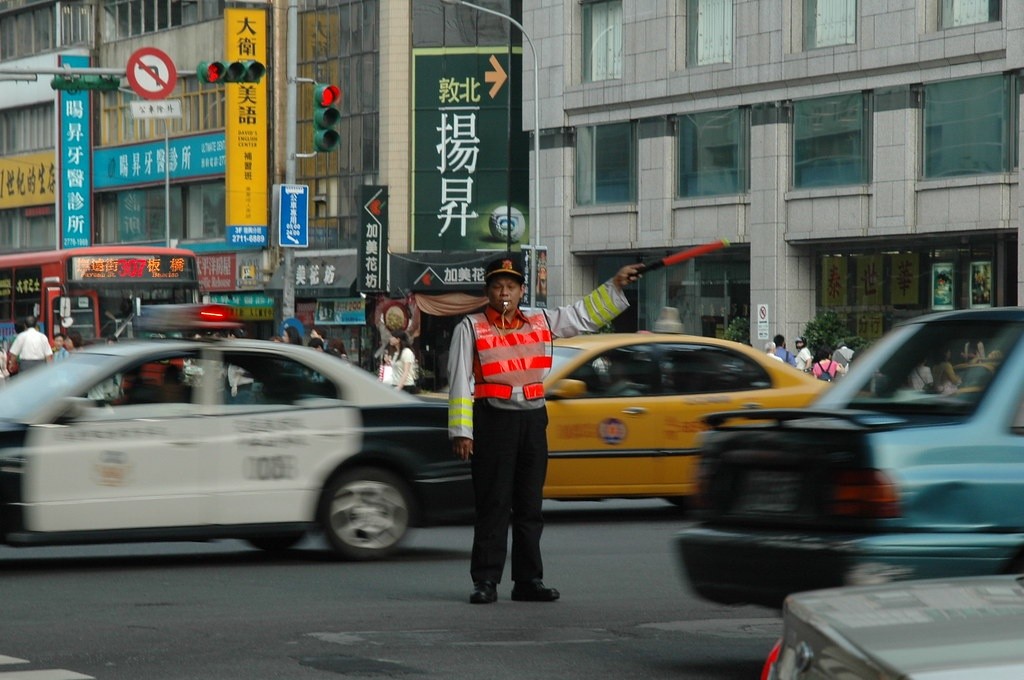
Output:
top-left (439, 0), bottom-right (539, 310)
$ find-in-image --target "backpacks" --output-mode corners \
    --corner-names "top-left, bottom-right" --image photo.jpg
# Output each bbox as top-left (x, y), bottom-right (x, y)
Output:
top-left (817, 359), bottom-right (832, 382)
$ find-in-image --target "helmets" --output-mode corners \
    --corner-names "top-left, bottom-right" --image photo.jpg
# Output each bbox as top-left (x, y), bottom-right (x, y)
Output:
top-left (795, 336), bottom-right (807, 349)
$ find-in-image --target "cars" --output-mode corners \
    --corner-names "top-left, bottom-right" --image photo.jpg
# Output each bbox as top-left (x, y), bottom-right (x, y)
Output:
top-left (673, 304), bottom-right (1024, 679)
top-left (542, 332), bottom-right (870, 501)
top-left (1, 339), bottom-right (478, 561)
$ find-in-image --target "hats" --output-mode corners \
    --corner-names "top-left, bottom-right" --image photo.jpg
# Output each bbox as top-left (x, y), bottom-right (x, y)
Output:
top-left (484, 258), bottom-right (526, 282)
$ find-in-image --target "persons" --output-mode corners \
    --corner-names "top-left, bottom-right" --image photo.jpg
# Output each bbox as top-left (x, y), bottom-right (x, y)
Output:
top-left (272, 327), bottom-right (421, 395)
top-left (0, 313), bottom-right (85, 381)
top-left (765, 335), bottom-right (962, 396)
top-left (593, 352), bottom-right (644, 397)
top-left (448, 252), bottom-right (646, 604)
top-left (159, 361), bottom-right (187, 402)
top-left (108, 368), bottom-right (154, 405)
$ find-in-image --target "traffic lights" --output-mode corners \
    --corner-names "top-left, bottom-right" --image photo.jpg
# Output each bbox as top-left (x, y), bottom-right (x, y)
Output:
top-left (197, 59), bottom-right (266, 85)
top-left (312, 83), bottom-right (343, 154)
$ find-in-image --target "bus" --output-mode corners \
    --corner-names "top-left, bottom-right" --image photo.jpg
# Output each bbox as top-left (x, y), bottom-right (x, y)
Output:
top-left (1, 245), bottom-right (206, 401)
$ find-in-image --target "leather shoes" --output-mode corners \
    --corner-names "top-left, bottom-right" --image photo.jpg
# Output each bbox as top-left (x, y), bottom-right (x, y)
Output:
top-left (511, 578), bottom-right (559, 601)
top-left (469, 579), bottom-right (498, 603)
